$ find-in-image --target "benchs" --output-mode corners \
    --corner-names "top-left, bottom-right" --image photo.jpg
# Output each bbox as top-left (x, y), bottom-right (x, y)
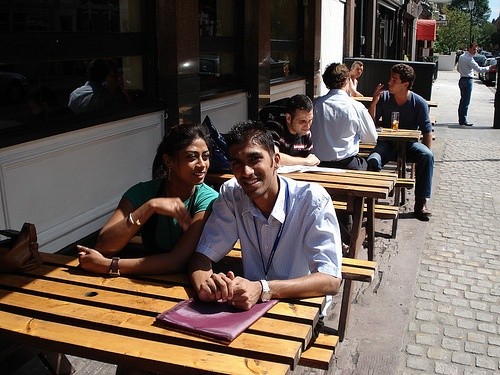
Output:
top-left (127, 228), bottom-right (378, 371)
top-left (332, 179), bottom-right (414, 239)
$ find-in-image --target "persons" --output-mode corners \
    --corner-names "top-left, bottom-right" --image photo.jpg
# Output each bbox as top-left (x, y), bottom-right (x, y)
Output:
top-left (76, 121), bottom-right (219, 278)
top-left (310, 62), bottom-right (379, 170)
top-left (346, 61), bottom-right (364, 97)
top-left (366, 63), bottom-right (433, 216)
top-left (68, 57), bottom-right (110, 114)
top-left (490, 55), bottom-right (500, 129)
top-left (270, 92), bottom-right (321, 166)
top-left (456, 43), bottom-right (489, 126)
top-left (187, 121), bottom-right (342, 352)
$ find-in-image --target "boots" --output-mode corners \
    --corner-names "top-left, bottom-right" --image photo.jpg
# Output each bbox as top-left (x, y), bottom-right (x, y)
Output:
top-left (414, 198), bottom-right (432, 217)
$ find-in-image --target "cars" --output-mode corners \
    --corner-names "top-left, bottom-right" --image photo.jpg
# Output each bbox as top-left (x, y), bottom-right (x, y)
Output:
top-left (473, 50), bottom-right (497, 85)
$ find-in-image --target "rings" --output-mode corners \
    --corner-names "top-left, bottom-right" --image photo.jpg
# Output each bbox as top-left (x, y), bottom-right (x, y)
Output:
top-left (85, 247), bottom-right (89, 253)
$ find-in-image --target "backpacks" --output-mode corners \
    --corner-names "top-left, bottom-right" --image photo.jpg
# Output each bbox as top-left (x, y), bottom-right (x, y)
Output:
top-left (259, 98), bottom-right (293, 148)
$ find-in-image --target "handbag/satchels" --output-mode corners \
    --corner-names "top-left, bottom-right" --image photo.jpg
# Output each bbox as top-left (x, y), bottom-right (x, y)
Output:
top-left (199, 115), bottom-right (231, 173)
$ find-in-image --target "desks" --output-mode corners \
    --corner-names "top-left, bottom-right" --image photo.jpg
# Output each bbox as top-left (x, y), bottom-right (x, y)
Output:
top-left (206, 166), bottom-right (399, 261)
top-left (377, 128), bottom-right (421, 204)
top-left (0, 251), bottom-right (327, 375)
top-left (354, 95), bottom-right (438, 109)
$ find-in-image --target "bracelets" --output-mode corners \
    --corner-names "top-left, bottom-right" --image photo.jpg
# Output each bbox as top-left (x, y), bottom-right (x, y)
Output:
top-left (128, 213), bottom-right (141, 226)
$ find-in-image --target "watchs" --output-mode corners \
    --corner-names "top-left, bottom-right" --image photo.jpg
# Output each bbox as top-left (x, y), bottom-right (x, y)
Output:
top-left (108, 257), bottom-right (121, 277)
top-left (259, 280), bottom-right (271, 302)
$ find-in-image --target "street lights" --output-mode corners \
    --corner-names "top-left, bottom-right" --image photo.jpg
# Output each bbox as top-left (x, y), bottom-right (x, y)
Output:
top-left (468, 0), bottom-right (475, 47)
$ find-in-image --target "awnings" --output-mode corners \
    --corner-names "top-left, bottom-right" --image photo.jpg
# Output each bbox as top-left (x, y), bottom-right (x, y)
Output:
top-left (416, 20), bottom-right (436, 40)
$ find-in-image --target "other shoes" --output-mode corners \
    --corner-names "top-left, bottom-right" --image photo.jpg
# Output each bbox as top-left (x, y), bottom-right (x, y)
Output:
top-left (461, 123), bottom-right (473, 127)
top-left (339, 212), bottom-right (353, 245)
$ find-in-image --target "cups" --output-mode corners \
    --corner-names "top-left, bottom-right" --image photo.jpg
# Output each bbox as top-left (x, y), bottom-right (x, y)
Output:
top-left (391, 112), bottom-right (400, 131)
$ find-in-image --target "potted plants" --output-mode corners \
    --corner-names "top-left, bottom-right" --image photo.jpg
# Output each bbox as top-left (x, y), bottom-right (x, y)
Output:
top-left (432, 6), bottom-right (470, 71)
